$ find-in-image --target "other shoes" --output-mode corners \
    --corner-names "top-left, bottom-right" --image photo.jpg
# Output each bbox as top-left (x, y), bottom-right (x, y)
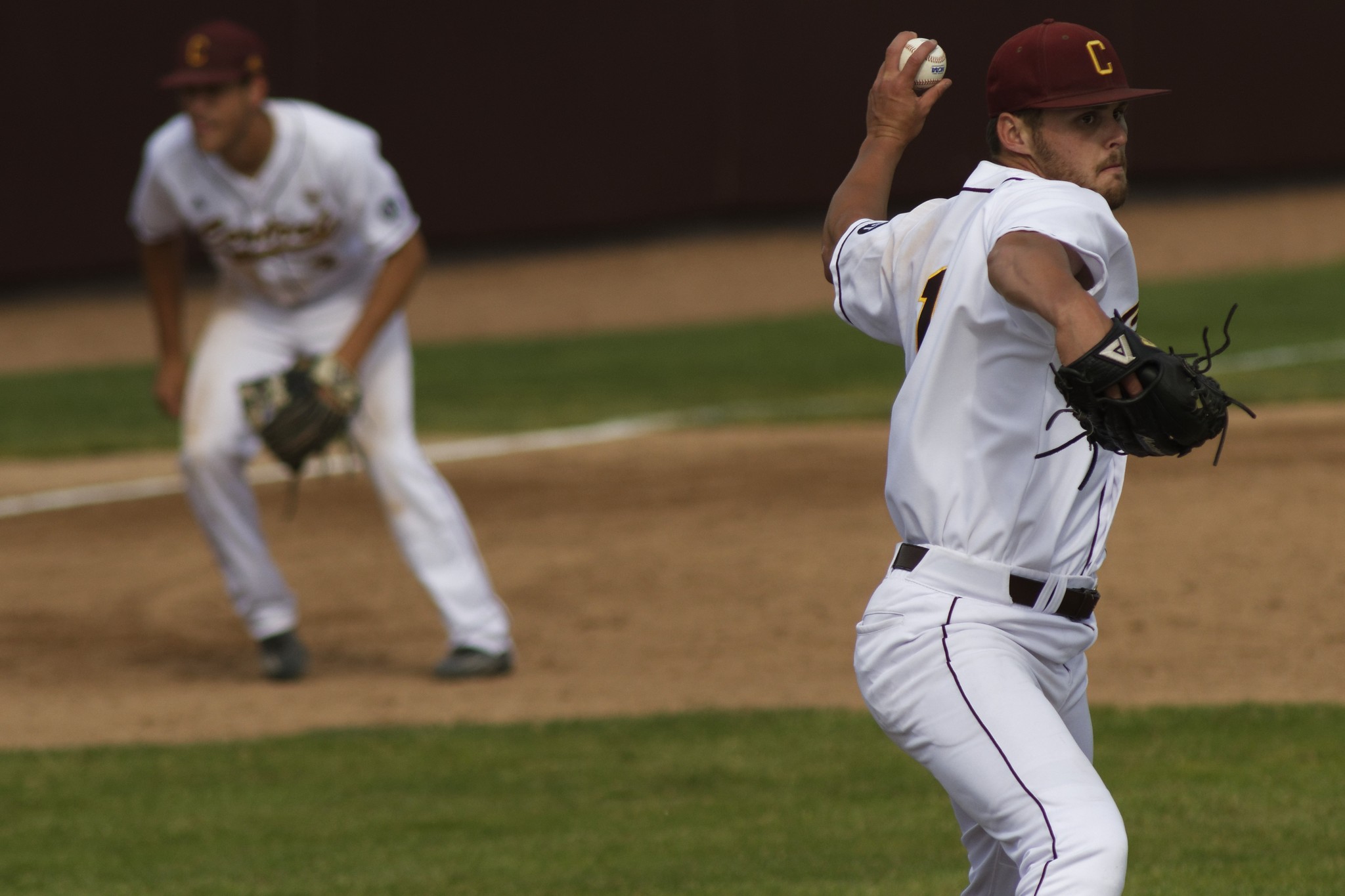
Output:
top-left (438, 651), bottom-right (512, 678)
top-left (256, 631), bottom-right (310, 679)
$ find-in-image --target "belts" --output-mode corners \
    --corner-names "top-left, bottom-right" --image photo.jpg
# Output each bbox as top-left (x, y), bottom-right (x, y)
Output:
top-left (891, 542), bottom-right (1101, 622)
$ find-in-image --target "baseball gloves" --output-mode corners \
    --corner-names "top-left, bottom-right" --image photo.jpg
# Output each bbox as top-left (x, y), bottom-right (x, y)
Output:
top-left (1055, 324), bottom-right (1227, 458)
top-left (241, 368), bottom-right (354, 473)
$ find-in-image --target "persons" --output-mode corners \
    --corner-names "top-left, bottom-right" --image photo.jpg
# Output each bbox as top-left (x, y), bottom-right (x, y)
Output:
top-left (131, 24), bottom-right (514, 685)
top-left (820, 19), bottom-right (1230, 896)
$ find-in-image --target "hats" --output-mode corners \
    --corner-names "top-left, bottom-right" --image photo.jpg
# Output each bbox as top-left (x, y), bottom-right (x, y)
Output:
top-left (158, 17), bottom-right (266, 90)
top-left (985, 18), bottom-right (1172, 115)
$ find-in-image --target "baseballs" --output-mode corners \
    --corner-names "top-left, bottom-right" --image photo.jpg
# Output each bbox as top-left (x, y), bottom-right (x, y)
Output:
top-left (898, 37), bottom-right (946, 90)
